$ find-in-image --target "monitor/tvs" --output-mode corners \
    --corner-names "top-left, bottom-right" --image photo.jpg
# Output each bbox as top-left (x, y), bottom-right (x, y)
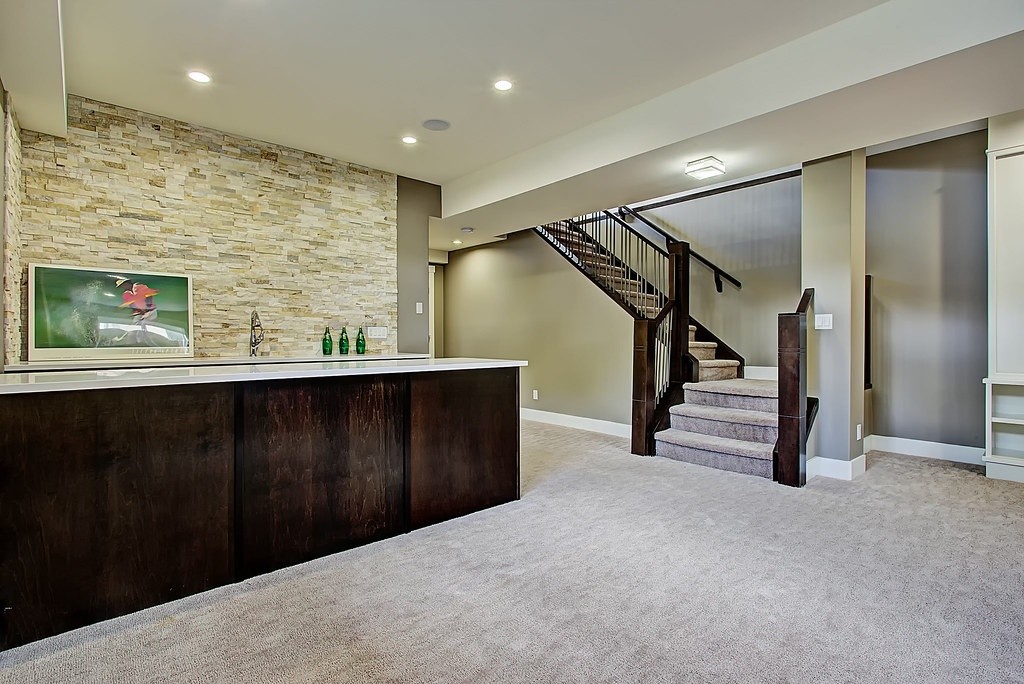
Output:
top-left (27, 263), bottom-right (195, 360)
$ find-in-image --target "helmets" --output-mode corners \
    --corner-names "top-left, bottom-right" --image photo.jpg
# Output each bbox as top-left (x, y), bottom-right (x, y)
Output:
top-left (115, 279), bottom-right (130, 288)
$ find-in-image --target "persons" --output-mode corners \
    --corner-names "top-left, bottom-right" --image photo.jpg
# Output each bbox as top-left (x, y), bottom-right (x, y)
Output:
top-left (116, 279), bottom-right (160, 347)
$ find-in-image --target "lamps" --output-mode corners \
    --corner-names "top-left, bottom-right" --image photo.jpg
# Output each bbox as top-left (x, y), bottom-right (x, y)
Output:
top-left (684, 156), bottom-right (726, 181)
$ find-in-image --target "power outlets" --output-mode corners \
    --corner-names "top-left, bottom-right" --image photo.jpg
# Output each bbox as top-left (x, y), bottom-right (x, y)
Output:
top-left (533, 390), bottom-right (538, 400)
top-left (856, 424), bottom-right (861, 441)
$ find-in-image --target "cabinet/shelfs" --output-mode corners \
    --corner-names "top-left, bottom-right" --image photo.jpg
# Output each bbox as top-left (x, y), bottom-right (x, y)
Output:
top-left (981, 378), bottom-right (1024, 484)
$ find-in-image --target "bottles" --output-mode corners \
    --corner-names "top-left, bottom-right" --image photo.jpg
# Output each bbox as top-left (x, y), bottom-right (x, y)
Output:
top-left (323, 326), bottom-right (332, 355)
top-left (339, 327), bottom-right (349, 354)
top-left (356, 326), bottom-right (365, 354)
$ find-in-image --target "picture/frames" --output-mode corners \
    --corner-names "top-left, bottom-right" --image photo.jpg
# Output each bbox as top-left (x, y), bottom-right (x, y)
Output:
top-left (27, 262), bottom-right (194, 362)
